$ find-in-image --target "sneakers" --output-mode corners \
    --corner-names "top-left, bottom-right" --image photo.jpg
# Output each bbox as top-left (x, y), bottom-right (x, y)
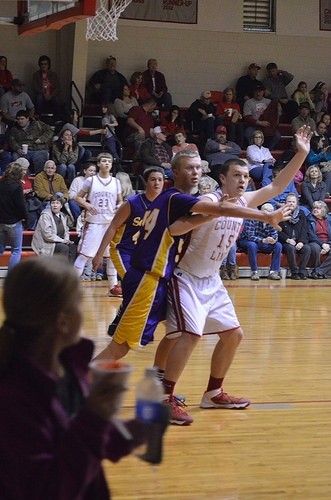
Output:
top-left (172, 394), bottom-right (185, 402)
top-left (199, 387), bottom-right (251, 409)
top-left (160, 396), bottom-right (194, 425)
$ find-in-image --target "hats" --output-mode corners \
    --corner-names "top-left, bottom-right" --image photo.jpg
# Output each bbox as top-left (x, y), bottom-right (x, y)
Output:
top-left (216, 125), bottom-right (227, 135)
top-left (200, 90), bottom-right (213, 99)
top-left (105, 55), bottom-right (117, 64)
top-left (153, 126), bottom-right (162, 135)
top-left (253, 83), bottom-right (265, 92)
top-left (9, 79), bottom-right (26, 87)
top-left (14, 158), bottom-right (30, 169)
top-left (248, 63), bottom-right (261, 70)
top-left (299, 102), bottom-right (311, 109)
top-left (15, 110), bottom-right (35, 122)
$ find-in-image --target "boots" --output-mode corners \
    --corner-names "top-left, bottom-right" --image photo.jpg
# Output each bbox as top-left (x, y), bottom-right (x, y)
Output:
top-left (229, 265), bottom-right (237, 280)
top-left (220, 266), bottom-right (230, 280)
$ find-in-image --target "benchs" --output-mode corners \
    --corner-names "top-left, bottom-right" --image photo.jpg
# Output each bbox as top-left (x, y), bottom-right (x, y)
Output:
top-left (0, 106), bottom-right (330, 269)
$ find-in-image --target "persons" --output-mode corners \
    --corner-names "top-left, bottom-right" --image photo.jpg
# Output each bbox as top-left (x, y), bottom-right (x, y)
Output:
top-left (0, 55), bottom-right (331, 426)
top-left (0, 256), bottom-right (172, 500)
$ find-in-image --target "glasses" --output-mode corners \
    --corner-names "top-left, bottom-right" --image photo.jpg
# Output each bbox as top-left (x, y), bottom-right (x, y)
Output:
top-left (252, 136), bottom-right (263, 138)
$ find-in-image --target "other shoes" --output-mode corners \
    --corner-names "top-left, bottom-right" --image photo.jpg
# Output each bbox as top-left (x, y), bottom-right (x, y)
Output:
top-left (250, 270), bottom-right (260, 280)
top-left (81, 275), bottom-right (103, 282)
top-left (298, 273), bottom-right (307, 280)
top-left (310, 271), bottom-right (324, 279)
top-left (106, 283), bottom-right (124, 297)
top-left (291, 273), bottom-right (301, 280)
top-left (267, 270), bottom-right (281, 280)
